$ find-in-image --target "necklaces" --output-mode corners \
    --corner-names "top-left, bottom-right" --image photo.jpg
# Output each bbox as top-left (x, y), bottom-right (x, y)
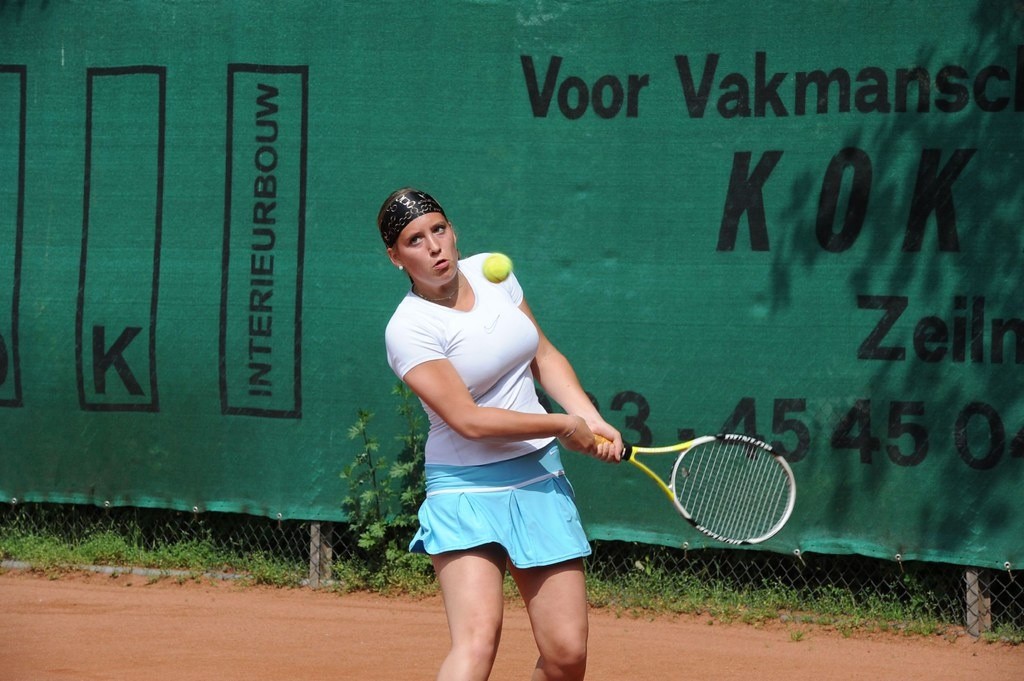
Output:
top-left (412, 270), bottom-right (464, 306)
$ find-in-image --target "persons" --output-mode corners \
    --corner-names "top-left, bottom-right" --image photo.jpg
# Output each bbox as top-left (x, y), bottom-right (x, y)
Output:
top-left (374, 182), bottom-right (632, 681)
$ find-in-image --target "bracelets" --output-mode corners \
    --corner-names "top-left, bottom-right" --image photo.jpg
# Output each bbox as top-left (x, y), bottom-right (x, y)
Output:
top-left (556, 414), bottom-right (579, 440)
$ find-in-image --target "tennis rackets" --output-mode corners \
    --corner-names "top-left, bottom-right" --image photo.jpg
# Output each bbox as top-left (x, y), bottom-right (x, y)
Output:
top-left (589, 431), bottom-right (797, 545)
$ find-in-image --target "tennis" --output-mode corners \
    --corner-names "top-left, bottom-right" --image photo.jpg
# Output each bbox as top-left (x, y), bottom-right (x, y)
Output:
top-left (482, 251), bottom-right (514, 284)
